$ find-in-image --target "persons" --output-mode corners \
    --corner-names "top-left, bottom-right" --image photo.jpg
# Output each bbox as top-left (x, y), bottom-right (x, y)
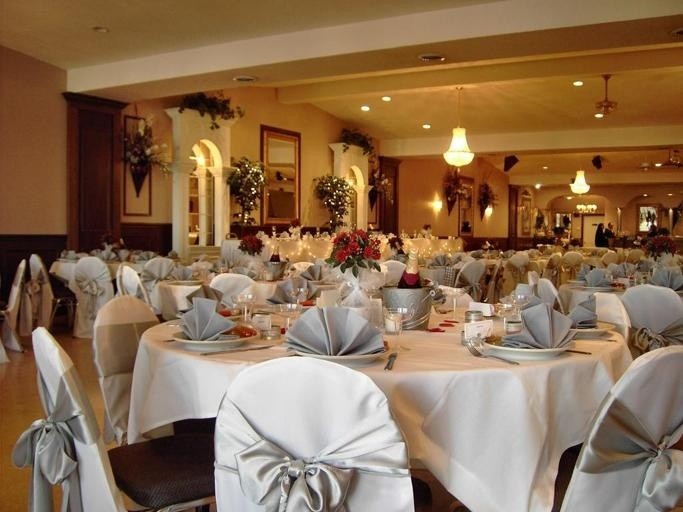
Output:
top-left (604, 222), bottom-right (618, 239)
top-left (641, 225), bottom-right (657, 246)
top-left (595, 223), bottom-right (609, 248)
top-left (540, 228), bottom-right (554, 245)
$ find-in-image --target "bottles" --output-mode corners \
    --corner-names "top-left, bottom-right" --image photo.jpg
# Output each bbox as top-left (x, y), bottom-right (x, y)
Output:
top-left (270, 242), bottom-right (279, 261)
top-left (397, 248), bottom-right (423, 289)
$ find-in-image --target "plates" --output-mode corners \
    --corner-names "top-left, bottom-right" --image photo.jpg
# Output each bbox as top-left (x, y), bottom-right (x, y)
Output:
top-left (59, 247), bottom-right (156, 265)
top-left (166, 279), bottom-right (203, 285)
top-left (567, 278), bottom-right (683, 294)
top-left (478, 340), bottom-right (569, 360)
top-left (570, 322), bottom-right (615, 338)
top-left (170, 327), bottom-right (258, 352)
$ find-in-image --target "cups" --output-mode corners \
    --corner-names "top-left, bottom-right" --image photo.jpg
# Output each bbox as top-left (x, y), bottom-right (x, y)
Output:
top-left (431, 278), bottom-right (533, 346)
top-left (192, 254), bottom-right (289, 283)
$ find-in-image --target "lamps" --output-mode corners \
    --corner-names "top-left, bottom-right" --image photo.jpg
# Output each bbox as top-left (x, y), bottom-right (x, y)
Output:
top-left (575, 202), bottom-right (597, 214)
top-left (594, 72), bottom-right (618, 114)
top-left (569, 169), bottom-right (590, 194)
top-left (444, 86), bottom-right (474, 167)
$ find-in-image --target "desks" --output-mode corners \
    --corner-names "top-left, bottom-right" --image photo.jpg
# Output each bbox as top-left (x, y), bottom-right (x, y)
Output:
top-left (140, 310), bottom-right (624, 512)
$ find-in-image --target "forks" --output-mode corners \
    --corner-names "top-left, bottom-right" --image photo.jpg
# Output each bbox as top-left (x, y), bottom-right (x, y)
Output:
top-left (461, 340), bottom-right (519, 365)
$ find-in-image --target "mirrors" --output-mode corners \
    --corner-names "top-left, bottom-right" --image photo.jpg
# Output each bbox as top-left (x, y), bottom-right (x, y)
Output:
top-left (458, 175), bottom-right (475, 237)
top-left (258, 123), bottom-right (302, 227)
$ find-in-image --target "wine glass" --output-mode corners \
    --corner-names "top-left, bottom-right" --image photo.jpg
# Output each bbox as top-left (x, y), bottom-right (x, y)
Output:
top-left (229, 274), bottom-right (413, 365)
top-left (270, 226), bottom-right (330, 241)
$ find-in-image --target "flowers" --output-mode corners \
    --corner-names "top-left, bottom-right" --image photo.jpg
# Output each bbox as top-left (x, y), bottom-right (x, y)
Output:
top-left (314, 174), bottom-right (354, 225)
top-left (477, 181), bottom-right (498, 215)
top-left (226, 150), bottom-right (270, 224)
top-left (368, 170), bottom-right (389, 195)
top-left (121, 111), bottom-right (171, 172)
top-left (441, 168), bottom-right (472, 203)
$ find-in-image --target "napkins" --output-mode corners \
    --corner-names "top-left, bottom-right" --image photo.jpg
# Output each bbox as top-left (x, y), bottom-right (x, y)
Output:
top-left (501, 300), bottom-right (579, 349)
top-left (282, 305), bottom-right (385, 356)
top-left (176, 297), bottom-right (237, 342)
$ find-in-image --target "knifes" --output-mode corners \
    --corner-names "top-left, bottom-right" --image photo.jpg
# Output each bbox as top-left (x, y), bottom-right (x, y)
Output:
top-left (383, 353), bottom-right (397, 371)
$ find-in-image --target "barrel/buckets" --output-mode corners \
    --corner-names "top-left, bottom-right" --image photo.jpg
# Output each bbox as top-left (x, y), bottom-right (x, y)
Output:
top-left (378, 277), bottom-right (434, 332)
top-left (264, 257), bottom-right (290, 281)
top-left (114, 248), bottom-right (130, 262)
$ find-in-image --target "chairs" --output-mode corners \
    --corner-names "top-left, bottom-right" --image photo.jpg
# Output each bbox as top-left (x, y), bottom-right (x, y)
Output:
top-left (22, 226), bottom-right (683, 337)
top-left (214, 356), bottom-right (414, 511)
top-left (560, 344), bottom-right (682, 511)
top-left (1, 259), bottom-right (26, 353)
top-left (31, 327), bottom-right (215, 512)
top-left (93, 296), bottom-right (175, 447)
